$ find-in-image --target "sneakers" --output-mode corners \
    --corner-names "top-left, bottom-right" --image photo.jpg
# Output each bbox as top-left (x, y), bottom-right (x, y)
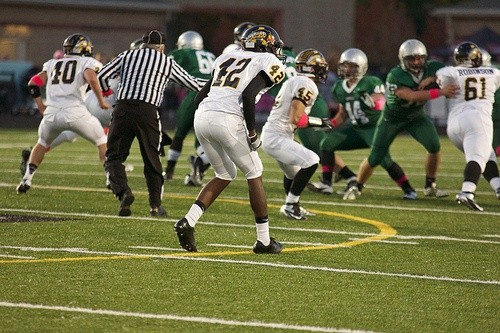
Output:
top-left (20, 150), bottom-right (31, 175)
top-left (16, 178), bottom-right (31, 193)
top-left (125, 163), bottom-right (134, 172)
top-left (424, 183), bottom-right (449, 197)
top-left (151, 207), bottom-right (167, 217)
top-left (456, 191), bottom-right (483, 211)
top-left (336, 174), bottom-right (366, 194)
top-left (253, 238), bottom-right (283, 254)
top-left (280, 202), bottom-right (307, 220)
top-left (174, 218), bottom-right (197, 252)
top-left (299, 206), bottom-right (316, 216)
top-left (119, 192), bottom-right (134, 216)
top-left (343, 186), bottom-right (361, 200)
top-left (403, 190), bottom-right (417, 200)
top-left (308, 180), bottom-right (333, 194)
top-left (185, 175), bottom-right (206, 187)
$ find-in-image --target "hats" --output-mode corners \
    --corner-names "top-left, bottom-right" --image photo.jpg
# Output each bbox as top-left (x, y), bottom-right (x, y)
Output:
top-left (143, 30), bottom-right (166, 44)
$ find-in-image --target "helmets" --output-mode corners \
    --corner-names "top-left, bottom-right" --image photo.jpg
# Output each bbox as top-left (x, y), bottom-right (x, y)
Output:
top-left (399, 40), bottom-right (427, 72)
top-left (234, 23), bottom-right (256, 46)
top-left (481, 50), bottom-right (491, 66)
top-left (294, 49), bottom-right (328, 83)
top-left (177, 31), bottom-right (203, 50)
top-left (455, 42), bottom-right (483, 67)
top-left (241, 25), bottom-right (286, 65)
top-left (130, 39), bottom-right (143, 49)
top-left (336, 49), bottom-right (368, 78)
top-left (63, 34), bottom-right (94, 57)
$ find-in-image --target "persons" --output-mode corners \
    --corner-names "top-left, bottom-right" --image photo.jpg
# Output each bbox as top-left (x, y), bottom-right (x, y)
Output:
top-left (303, 47), bottom-right (420, 200)
top-left (16, 33), bottom-right (133, 194)
top-left (260, 49), bottom-right (334, 220)
top-left (436, 42), bottom-right (499, 212)
top-left (342, 40), bottom-right (461, 200)
top-left (165, 31), bottom-right (215, 183)
top-left (174, 25), bottom-right (283, 254)
top-left (95, 30), bottom-right (209, 218)
top-left (231, 23), bottom-right (356, 193)
